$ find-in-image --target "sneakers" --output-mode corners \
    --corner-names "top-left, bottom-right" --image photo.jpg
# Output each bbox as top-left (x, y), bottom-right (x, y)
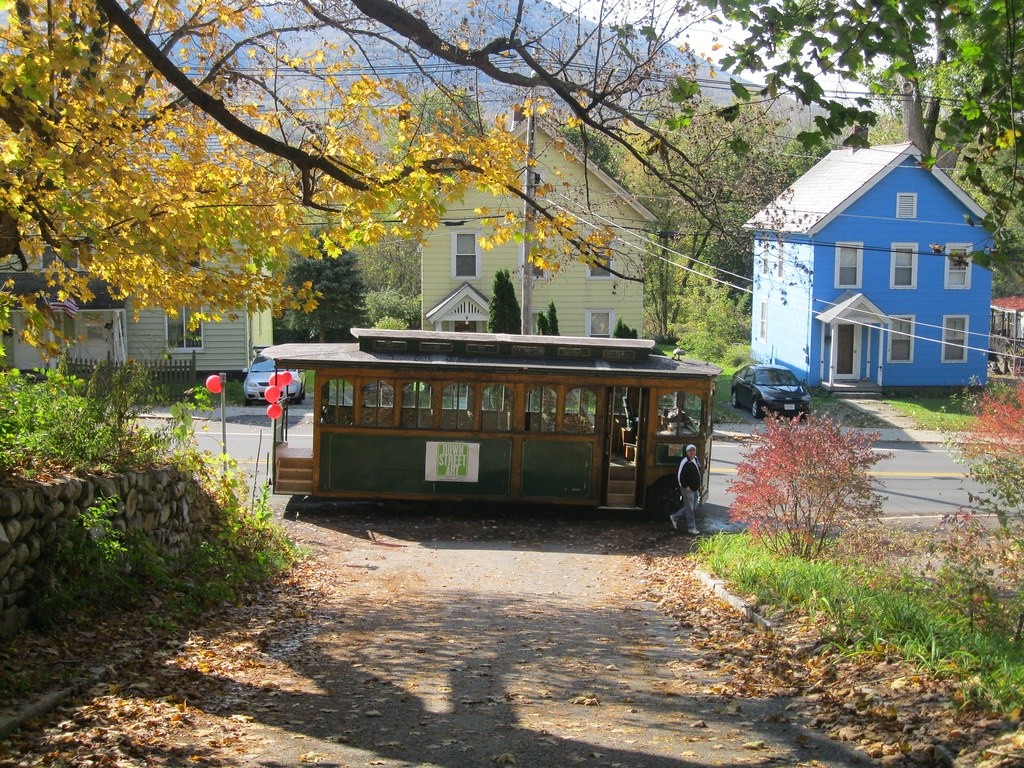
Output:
top-left (670, 515), bottom-right (677, 530)
top-left (688, 529), bottom-right (700, 535)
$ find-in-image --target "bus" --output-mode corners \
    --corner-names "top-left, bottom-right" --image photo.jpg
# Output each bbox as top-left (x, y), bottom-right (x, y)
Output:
top-left (257, 330), bottom-right (725, 530)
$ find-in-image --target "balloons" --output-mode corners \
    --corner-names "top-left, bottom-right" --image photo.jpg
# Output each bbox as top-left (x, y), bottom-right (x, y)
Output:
top-left (268, 374), bottom-right (284, 388)
top-left (205, 375), bottom-right (221, 393)
top-left (278, 371), bottom-right (293, 386)
top-left (266, 404), bottom-right (282, 419)
top-left (265, 385), bottom-right (280, 404)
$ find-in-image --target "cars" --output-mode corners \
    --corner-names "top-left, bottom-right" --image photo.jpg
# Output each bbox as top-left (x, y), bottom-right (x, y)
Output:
top-left (729, 362), bottom-right (811, 420)
top-left (240, 350), bottom-right (307, 403)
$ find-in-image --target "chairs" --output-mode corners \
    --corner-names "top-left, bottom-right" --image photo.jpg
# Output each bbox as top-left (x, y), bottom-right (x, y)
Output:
top-left (622, 396), bottom-right (647, 435)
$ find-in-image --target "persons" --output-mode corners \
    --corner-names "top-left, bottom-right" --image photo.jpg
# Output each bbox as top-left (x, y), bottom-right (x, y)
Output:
top-left (670, 444), bottom-right (701, 534)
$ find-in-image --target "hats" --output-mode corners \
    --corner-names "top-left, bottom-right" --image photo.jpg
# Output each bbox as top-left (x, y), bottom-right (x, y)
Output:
top-left (686, 444), bottom-right (696, 451)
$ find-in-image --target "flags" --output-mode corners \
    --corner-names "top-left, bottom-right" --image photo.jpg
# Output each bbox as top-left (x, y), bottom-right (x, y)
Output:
top-left (42, 294), bottom-right (78, 320)
